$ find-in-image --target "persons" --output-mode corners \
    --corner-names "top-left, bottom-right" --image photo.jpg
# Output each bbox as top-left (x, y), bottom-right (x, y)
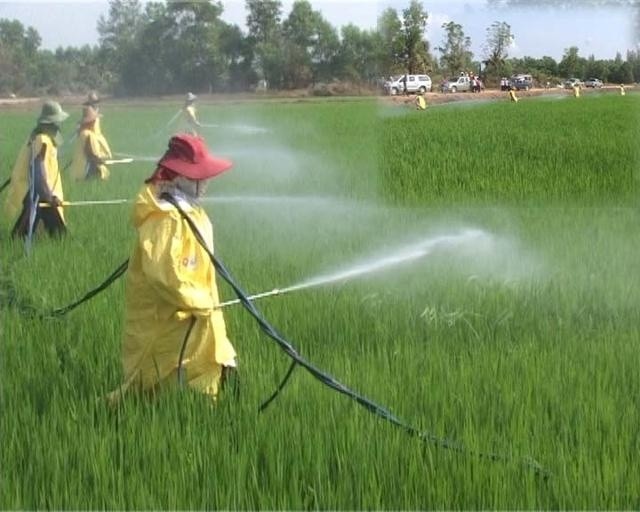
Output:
top-left (471, 77), bottom-right (481, 93)
top-left (114, 133), bottom-right (242, 416)
top-left (182, 92), bottom-right (202, 135)
top-left (509, 88), bottom-right (518, 102)
top-left (573, 86), bottom-right (580, 98)
top-left (460, 71), bottom-right (473, 77)
top-left (415, 92), bottom-right (426, 110)
top-left (620, 84), bottom-right (625, 96)
top-left (403, 75), bottom-right (410, 96)
top-left (381, 76), bottom-right (393, 95)
top-left (500, 78), bottom-right (510, 91)
top-left (74, 91), bottom-right (111, 183)
top-left (10, 99), bottom-right (67, 242)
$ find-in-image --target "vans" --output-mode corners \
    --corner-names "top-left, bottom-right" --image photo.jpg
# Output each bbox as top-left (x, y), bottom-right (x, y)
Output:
top-left (383, 74), bottom-right (432, 95)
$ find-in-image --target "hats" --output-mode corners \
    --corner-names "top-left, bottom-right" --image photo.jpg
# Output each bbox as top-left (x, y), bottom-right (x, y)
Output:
top-left (158, 132), bottom-right (234, 181)
top-left (82, 93), bottom-right (100, 104)
top-left (37, 100), bottom-right (69, 124)
top-left (76, 105), bottom-right (103, 124)
top-left (186, 92), bottom-right (196, 100)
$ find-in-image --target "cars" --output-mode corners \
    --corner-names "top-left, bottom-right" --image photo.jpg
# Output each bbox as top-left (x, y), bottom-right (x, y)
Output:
top-left (565, 78), bottom-right (581, 89)
top-left (586, 78), bottom-right (603, 89)
top-left (444, 76), bottom-right (472, 92)
